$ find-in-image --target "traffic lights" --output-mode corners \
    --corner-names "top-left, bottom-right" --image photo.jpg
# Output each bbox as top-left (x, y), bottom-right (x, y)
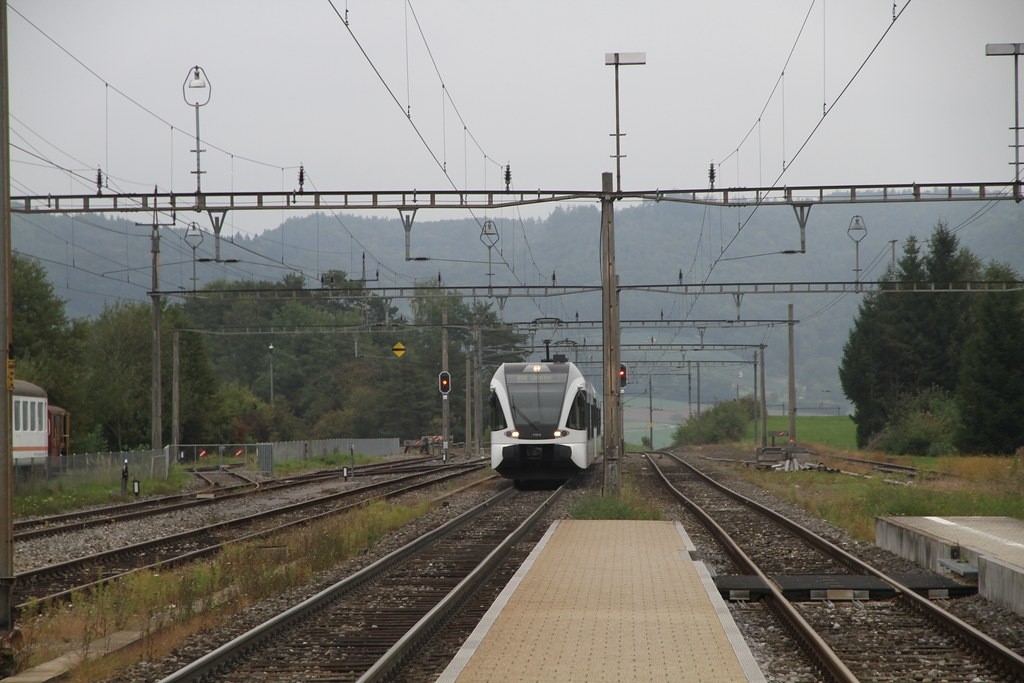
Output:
top-left (439, 371), bottom-right (451, 394)
top-left (620, 363), bottom-right (628, 389)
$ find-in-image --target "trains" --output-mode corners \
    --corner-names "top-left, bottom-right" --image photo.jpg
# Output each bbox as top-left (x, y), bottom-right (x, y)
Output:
top-left (489, 359), bottom-right (604, 487)
top-left (12, 379), bottom-right (50, 477)
